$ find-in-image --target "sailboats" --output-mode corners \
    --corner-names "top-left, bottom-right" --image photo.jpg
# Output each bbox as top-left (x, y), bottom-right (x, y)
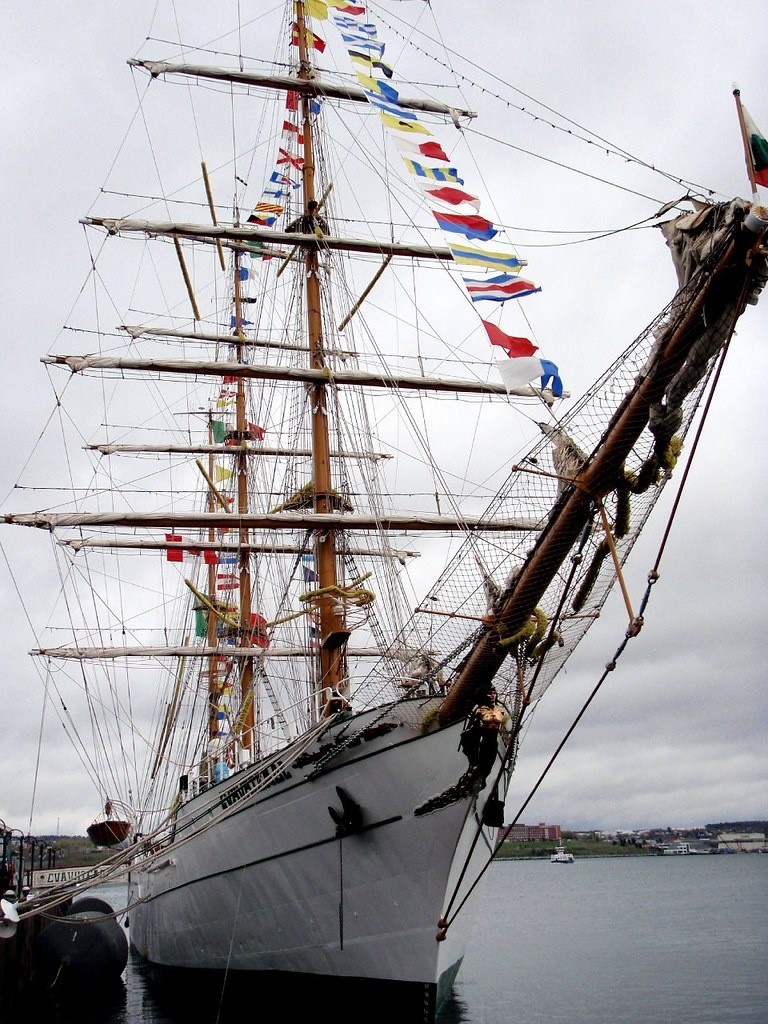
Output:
top-left (0, 0), bottom-right (768, 1024)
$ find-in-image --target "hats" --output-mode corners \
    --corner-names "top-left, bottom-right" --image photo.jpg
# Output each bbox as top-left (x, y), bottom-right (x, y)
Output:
top-left (4, 889), bottom-right (15, 895)
top-left (22, 886), bottom-right (31, 891)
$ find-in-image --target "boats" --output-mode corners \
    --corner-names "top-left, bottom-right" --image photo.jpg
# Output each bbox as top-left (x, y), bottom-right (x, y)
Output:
top-left (551, 840), bottom-right (575, 864)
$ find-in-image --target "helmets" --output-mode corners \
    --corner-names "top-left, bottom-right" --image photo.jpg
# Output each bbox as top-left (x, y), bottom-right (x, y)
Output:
top-left (483, 685), bottom-right (498, 704)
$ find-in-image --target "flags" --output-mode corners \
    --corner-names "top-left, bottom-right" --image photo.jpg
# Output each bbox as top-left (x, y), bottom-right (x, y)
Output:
top-left (481, 318), bottom-right (540, 357)
top-left (419, 183), bottom-right (481, 212)
top-left (363, 88), bottom-right (418, 120)
top-left (291, 22), bottom-right (326, 52)
top-left (450, 243), bottom-right (523, 273)
top-left (327, 0), bottom-right (385, 61)
top-left (282, 121), bottom-right (305, 144)
top-left (285, 88), bottom-right (321, 115)
top-left (495, 358), bottom-right (563, 398)
top-left (735, 100), bottom-right (768, 191)
top-left (379, 114), bottom-right (435, 137)
top-left (403, 158), bottom-right (465, 186)
top-left (433, 210), bottom-right (500, 241)
top-left (214, 146), bottom-right (304, 405)
top-left (294, 1), bottom-right (329, 22)
top-left (348, 50), bottom-right (394, 77)
top-left (389, 136), bottom-right (451, 162)
top-left (462, 275), bottom-right (542, 302)
top-left (355, 70), bottom-right (399, 105)
top-left (165, 419), bottom-right (268, 720)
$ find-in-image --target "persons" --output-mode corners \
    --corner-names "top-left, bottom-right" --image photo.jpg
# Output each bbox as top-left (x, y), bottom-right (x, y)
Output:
top-left (207, 731), bottom-right (228, 778)
top-left (9, 865), bottom-right (19, 895)
